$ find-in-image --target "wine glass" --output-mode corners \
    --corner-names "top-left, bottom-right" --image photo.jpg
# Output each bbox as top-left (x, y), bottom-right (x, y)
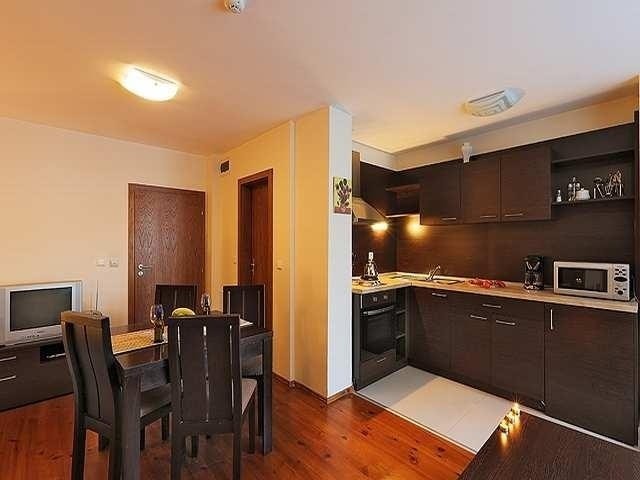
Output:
top-left (199, 292), bottom-right (211, 315)
top-left (148, 303), bottom-right (165, 343)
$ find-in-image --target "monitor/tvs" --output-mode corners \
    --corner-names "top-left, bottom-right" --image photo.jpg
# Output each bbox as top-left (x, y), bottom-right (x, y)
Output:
top-left (0, 280), bottom-right (84, 346)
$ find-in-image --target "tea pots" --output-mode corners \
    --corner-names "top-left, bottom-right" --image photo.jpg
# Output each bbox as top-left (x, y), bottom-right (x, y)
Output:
top-left (361, 259), bottom-right (379, 281)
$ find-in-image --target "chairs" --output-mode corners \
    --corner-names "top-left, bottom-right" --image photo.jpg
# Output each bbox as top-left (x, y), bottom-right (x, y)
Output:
top-left (154, 283), bottom-right (202, 439)
top-left (59, 310), bottom-right (174, 479)
top-left (163, 313), bottom-right (257, 478)
top-left (223, 282), bottom-right (266, 438)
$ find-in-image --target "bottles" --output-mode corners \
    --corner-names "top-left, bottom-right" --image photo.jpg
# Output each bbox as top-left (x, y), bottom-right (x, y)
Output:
top-left (555, 170), bottom-right (624, 203)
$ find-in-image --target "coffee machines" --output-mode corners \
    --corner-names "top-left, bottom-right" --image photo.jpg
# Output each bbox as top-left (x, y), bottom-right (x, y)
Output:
top-left (521, 254), bottom-right (545, 292)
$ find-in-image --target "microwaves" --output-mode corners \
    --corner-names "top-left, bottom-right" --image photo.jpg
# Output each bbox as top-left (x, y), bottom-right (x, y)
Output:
top-left (553, 259), bottom-right (634, 303)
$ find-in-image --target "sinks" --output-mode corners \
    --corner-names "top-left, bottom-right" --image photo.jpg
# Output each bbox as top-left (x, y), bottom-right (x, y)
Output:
top-left (390, 275), bottom-right (428, 280)
top-left (418, 277), bottom-right (465, 285)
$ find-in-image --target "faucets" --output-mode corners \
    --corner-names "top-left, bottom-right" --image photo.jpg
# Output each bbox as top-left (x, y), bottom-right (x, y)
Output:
top-left (427, 265), bottom-right (441, 281)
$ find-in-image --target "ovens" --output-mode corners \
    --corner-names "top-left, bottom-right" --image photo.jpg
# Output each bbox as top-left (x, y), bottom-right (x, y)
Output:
top-left (354, 288), bottom-right (397, 388)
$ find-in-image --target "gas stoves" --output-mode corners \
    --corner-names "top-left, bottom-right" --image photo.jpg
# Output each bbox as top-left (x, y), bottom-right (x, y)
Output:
top-left (353, 275), bottom-right (412, 294)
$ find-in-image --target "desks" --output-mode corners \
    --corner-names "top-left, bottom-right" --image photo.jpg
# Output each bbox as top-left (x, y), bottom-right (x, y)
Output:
top-left (456, 408), bottom-right (639, 479)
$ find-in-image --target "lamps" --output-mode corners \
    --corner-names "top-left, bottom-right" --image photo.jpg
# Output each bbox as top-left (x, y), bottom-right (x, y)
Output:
top-left (462, 87), bottom-right (526, 118)
top-left (117, 63), bottom-right (179, 102)
top-left (460, 141), bottom-right (473, 163)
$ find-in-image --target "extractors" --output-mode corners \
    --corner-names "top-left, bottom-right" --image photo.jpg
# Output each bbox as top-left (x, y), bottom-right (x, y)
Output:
top-left (352, 151), bottom-right (391, 226)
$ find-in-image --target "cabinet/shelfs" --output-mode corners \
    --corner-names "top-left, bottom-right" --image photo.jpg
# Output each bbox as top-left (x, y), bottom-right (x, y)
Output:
top-left (386, 180), bottom-right (421, 218)
top-left (450, 290), bottom-right (545, 401)
top-left (411, 286), bottom-right (451, 375)
top-left (553, 148), bottom-right (635, 205)
top-left (1, 338), bottom-right (75, 410)
top-left (419, 160), bottom-right (462, 228)
top-left (463, 146), bottom-right (554, 224)
top-left (547, 308), bottom-right (635, 447)
